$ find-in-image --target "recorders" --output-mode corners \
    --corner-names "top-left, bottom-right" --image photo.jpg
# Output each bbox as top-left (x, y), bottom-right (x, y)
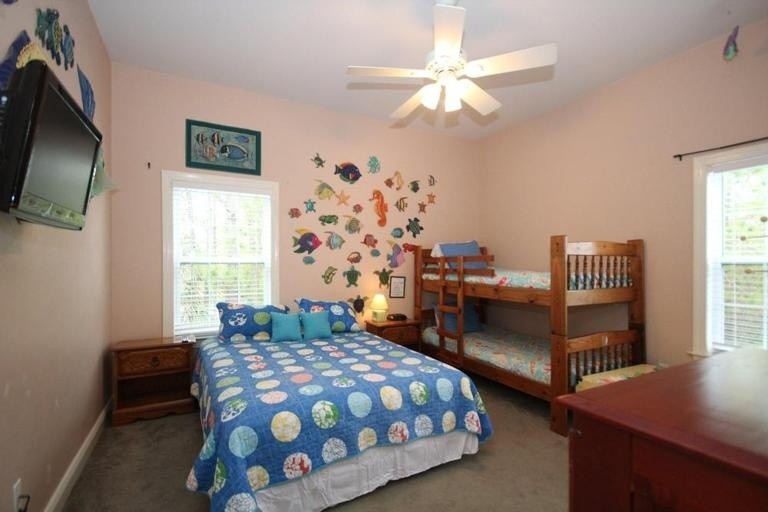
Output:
top-left (387, 313), bottom-right (407, 321)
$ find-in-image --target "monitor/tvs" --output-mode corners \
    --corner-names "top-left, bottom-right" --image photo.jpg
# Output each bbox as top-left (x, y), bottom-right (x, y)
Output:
top-left (0, 59), bottom-right (102, 231)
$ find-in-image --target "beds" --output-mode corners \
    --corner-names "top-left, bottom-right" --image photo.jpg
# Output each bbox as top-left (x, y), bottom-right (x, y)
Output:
top-left (414, 235), bottom-right (646, 438)
top-left (194, 331), bottom-right (479, 512)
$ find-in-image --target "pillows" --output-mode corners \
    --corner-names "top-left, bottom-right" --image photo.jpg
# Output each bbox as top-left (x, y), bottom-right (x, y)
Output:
top-left (215, 297), bottom-right (359, 343)
top-left (432, 302), bottom-right (483, 335)
top-left (430, 241), bottom-right (487, 275)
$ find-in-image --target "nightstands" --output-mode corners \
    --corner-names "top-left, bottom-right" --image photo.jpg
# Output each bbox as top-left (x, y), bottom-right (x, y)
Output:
top-left (105, 334), bottom-right (196, 427)
top-left (363, 318), bottom-right (422, 353)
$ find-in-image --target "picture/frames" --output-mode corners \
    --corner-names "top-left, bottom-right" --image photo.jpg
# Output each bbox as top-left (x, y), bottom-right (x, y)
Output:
top-left (185, 118), bottom-right (262, 177)
top-left (389, 276), bottom-right (406, 299)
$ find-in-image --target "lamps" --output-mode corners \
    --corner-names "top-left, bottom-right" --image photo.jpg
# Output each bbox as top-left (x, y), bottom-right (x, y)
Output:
top-left (370, 295), bottom-right (388, 322)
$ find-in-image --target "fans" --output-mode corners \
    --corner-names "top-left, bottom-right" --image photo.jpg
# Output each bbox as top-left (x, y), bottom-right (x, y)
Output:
top-left (341, 1), bottom-right (558, 122)
